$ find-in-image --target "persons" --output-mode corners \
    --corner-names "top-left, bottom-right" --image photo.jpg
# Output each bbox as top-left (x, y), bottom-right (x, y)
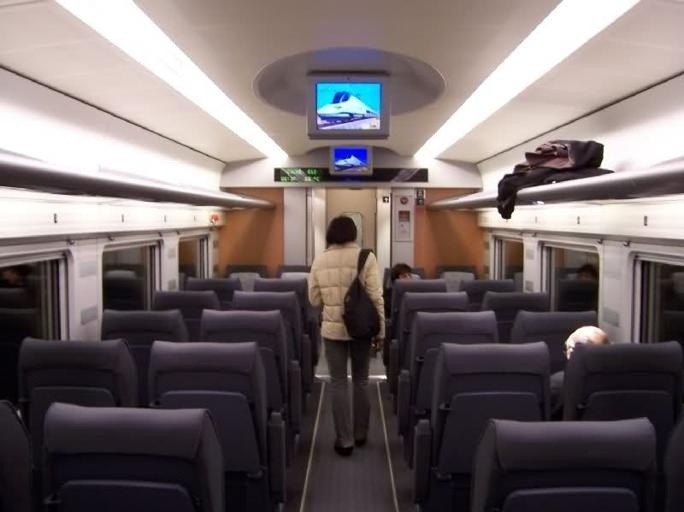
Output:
top-left (306, 214), bottom-right (386, 458)
top-left (383, 262), bottom-right (414, 319)
top-left (545, 325), bottom-right (611, 418)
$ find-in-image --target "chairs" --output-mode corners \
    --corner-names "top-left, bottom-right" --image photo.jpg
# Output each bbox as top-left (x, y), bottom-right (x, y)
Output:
top-left (381, 263), bottom-right (684, 511)
top-left (0, 263), bottom-right (323, 511)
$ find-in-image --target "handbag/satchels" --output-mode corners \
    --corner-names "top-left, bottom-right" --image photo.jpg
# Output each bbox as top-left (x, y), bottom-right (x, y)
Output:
top-left (342, 278), bottom-right (380, 340)
top-left (524, 140), bottom-right (603, 170)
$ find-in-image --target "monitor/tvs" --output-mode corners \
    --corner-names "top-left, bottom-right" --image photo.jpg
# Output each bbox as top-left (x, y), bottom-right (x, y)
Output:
top-left (330, 146), bottom-right (374, 176)
top-left (308, 75), bottom-right (390, 139)
